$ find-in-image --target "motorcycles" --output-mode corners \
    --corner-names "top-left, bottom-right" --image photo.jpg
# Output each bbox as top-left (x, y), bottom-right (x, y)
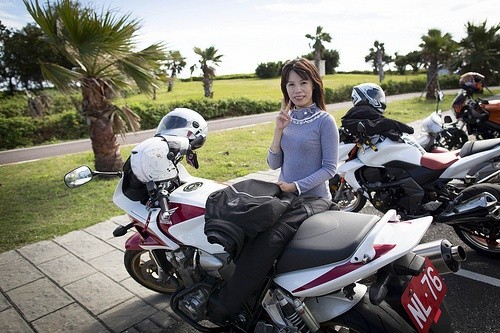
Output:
top-left (328, 82), bottom-right (500, 253)
top-left (63, 107), bottom-right (466, 333)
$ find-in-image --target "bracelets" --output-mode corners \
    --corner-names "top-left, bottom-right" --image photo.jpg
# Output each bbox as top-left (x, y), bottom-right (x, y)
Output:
top-left (271, 142), bottom-right (281, 149)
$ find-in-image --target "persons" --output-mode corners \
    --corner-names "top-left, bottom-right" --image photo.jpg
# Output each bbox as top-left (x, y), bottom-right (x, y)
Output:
top-left (266, 57), bottom-right (339, 210)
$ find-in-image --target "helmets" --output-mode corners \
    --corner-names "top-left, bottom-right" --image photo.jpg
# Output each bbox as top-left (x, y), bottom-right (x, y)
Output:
top-left (459, 72), bottom-right (485, 93)
top-left (156, 108), bottom-right (208, 150)
top-left (351, 82), bottom-right (386, 114)
